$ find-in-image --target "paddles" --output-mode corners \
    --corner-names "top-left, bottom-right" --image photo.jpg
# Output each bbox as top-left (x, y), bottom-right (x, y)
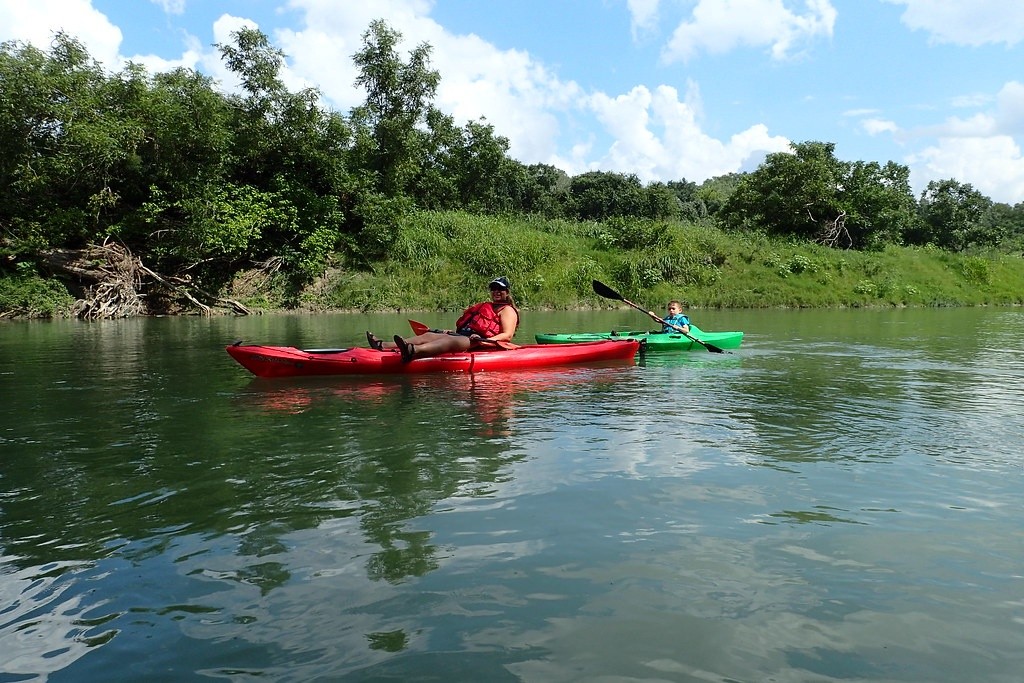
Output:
top-left (592, 279), bottom-right (734, 354)
top-left (407, 317), bottom-right (522, 350)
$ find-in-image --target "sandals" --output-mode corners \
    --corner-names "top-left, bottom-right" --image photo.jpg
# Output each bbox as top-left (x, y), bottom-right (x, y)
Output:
top-left (394, 335), bottom-right (414, 362)
top-left (366, 331), bottom-right (383, 349)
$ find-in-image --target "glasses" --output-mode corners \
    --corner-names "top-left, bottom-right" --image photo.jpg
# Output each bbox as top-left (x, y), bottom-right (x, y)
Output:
top-left (490, 285), bottom-right (506, 290)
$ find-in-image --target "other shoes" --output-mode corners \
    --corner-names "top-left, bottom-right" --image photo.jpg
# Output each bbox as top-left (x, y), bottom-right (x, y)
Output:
top-left (612, 331), bottom-right (618, 336)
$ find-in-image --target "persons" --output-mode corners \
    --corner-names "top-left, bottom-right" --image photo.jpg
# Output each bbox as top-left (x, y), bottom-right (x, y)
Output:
top-left (611, 300), bottom-right (690, 336)
top-left (366, 277), bottom-right (519, 363)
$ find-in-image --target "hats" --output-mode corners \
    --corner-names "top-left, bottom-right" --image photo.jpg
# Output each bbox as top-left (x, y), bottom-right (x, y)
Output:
top-left (489, 276), bottom-right (510, 289)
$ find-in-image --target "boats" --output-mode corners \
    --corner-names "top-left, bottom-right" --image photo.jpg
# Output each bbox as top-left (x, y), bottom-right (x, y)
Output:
top-left (224, 338), bottom-right (640, 378)
top-left (535, 324), bottom-right (744, 352)
